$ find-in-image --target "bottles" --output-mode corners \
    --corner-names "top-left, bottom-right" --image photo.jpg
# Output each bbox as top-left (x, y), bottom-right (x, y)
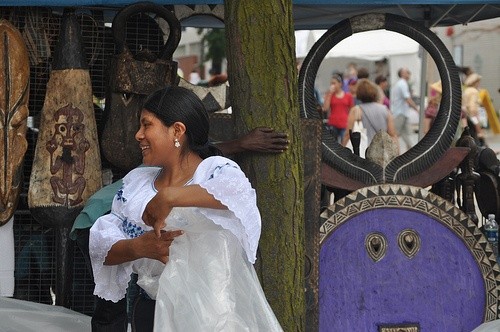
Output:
top-left (485, 214), bottom-right (499, 257)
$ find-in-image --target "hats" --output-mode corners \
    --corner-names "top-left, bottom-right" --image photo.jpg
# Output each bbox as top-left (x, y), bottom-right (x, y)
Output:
top-left (464, 73), bottom-right (482, 86)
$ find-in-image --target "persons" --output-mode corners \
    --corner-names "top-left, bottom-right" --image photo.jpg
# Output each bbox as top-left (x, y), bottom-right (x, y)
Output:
top-left (298, 63), bottom-right (500, 216)
top-left (90, 87), bottom-right (284, 332)
top-left (68, 127), bottom-right (293, 332)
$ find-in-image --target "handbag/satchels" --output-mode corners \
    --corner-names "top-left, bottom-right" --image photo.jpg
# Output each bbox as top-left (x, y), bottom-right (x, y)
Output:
top-left (344, 106), bottom-right (368, 159)
